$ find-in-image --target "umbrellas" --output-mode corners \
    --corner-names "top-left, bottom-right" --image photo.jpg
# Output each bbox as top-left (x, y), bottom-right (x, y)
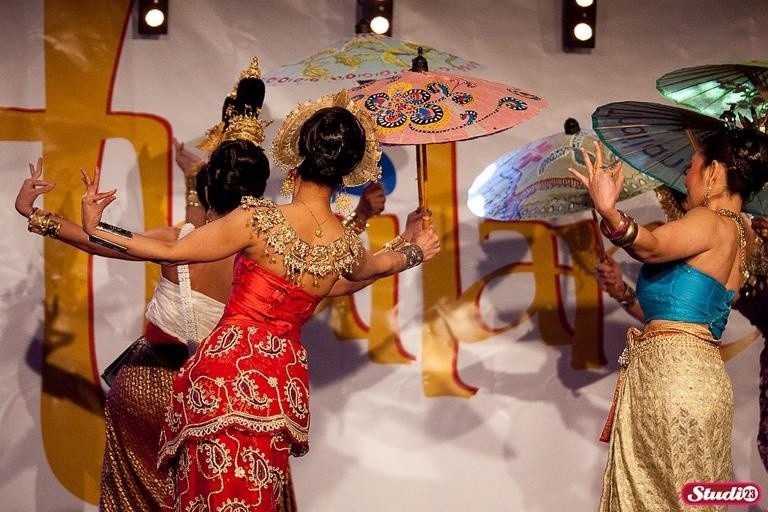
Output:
top-left (347, 44), bottom-right (548, 231)
top-left (467, 116), bottom-right (664, 298)
top-left (260, 18), bottom-right (486, 216)
top-left (656, 56), bottom-right (768, 131)
top-left (592, 100), bottom-right (768, 221)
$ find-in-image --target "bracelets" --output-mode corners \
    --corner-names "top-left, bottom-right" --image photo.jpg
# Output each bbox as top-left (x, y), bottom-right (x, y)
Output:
top-left (346, 217), bottom-right (368, 235)
top-left (28, 207), bottom-right (65, 240)
top-left (184, 173), bottom-right (202, 206)
top-left (610, 216), bottom-right (638, 248)
top-left (600, 209), bottom-right (629, 240)
top-left (88, 222), bottom-right (133, 254)
top-left (620, 282), bottom-right (636, 311)
top-left (384, 234), bottom-right (406, 251)
top-left (395, 244), bottom-right (423, 270)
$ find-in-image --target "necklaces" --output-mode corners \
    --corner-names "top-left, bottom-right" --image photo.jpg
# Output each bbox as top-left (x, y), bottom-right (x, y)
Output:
top-left (297, 199), bottom-right (333, 235)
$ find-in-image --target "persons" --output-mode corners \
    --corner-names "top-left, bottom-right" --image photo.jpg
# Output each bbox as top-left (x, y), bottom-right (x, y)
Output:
top-left (567, 130), bottom-right (767, 512)
top-left (593, 185), bottom-right (768, 475)
top-left (85, 87), bottom-right (442, 512)
top-left (15, 56), bottom-right (432, 512)
top-left (169, 57), bottom-right (386, 235)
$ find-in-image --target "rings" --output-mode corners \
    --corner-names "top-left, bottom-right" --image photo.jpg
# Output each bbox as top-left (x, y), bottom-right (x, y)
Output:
top-left (593, 160), bottom-right (599, 176)
